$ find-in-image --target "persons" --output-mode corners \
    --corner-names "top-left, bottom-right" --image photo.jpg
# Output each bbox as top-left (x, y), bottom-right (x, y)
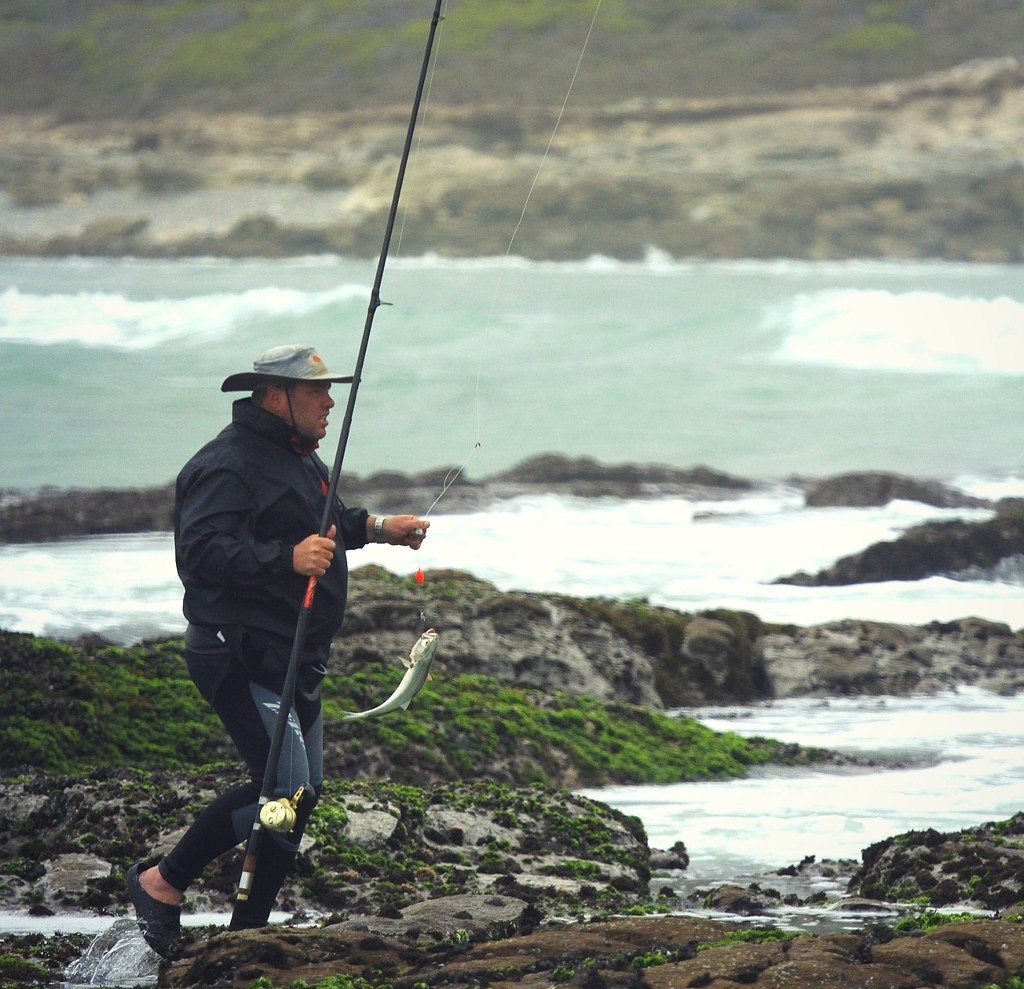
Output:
top-left (124, 345), bottom-right (430, 963)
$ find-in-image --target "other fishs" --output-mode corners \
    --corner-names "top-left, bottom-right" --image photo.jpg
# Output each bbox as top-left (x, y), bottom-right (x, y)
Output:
top-left (341, 628), bottom-right (440, 721)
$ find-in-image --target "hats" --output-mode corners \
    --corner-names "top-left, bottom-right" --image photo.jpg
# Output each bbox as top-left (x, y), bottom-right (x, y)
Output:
top-left (221, 344), bottom-right (361, 393)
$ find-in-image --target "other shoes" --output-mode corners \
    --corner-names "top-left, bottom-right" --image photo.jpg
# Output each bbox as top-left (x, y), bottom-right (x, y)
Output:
top-left (126, 862), bottom-right (183, 961)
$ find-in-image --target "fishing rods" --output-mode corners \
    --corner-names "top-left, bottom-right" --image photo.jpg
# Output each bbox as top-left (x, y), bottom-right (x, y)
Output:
top-left (235, 1), bottom-right (444, 915)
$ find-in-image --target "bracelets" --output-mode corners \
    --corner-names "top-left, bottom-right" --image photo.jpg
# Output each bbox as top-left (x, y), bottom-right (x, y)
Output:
top-left (374, 517), bottom-right (386, 544)
